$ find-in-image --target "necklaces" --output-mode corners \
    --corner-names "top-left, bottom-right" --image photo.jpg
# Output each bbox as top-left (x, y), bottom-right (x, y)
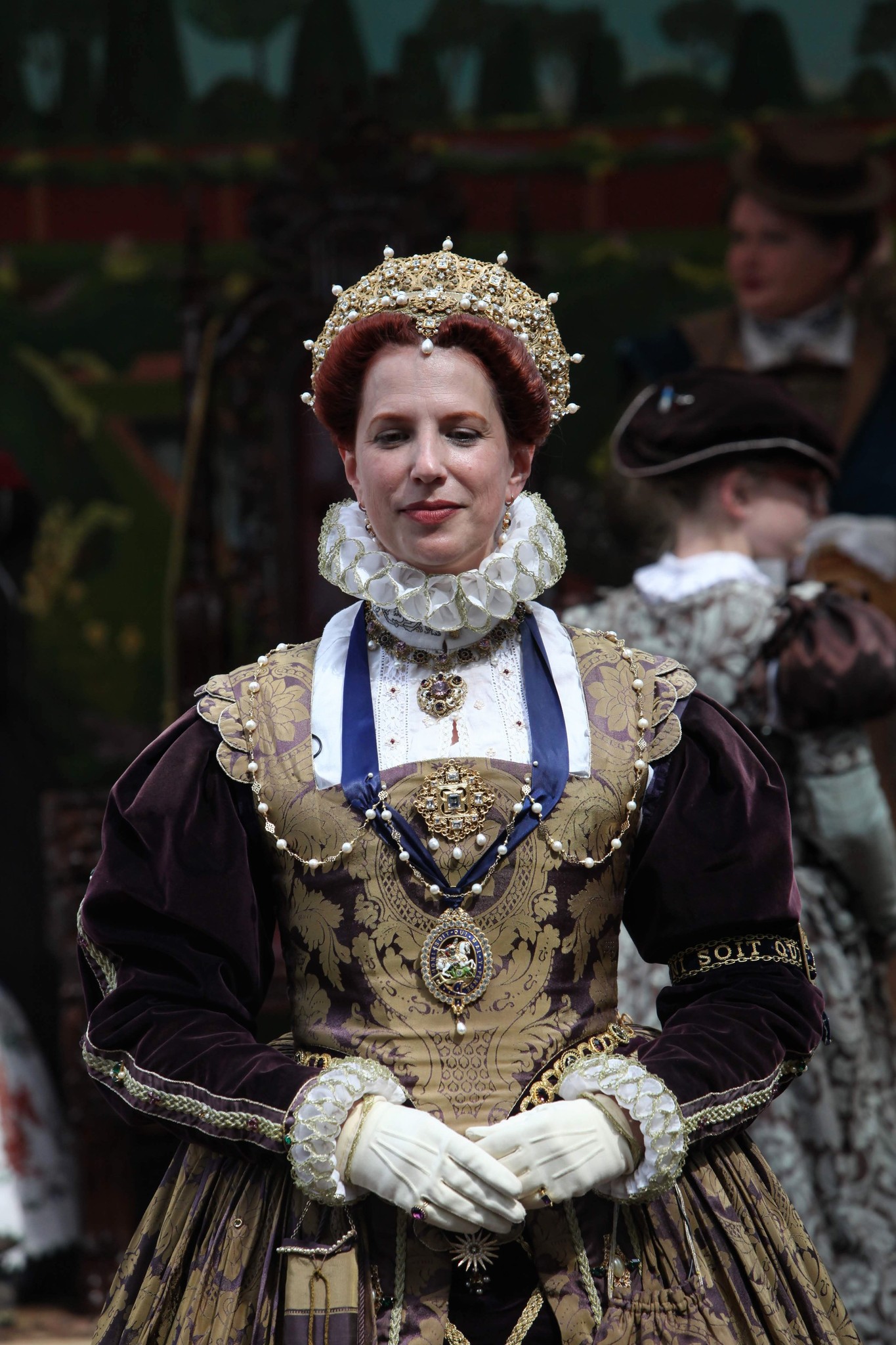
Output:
top-left (363, 601), bottom-right (527, 719)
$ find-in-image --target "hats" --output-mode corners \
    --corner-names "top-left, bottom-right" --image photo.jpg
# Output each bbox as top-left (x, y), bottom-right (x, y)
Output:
top-left (303, 236), bottom-right (585, 447)
top-left (727, 126), bottom-right (890, 219)
top-left (791, 513), bottom-right (895, 585)
top-left (610, 385), bottom-right (843, 475)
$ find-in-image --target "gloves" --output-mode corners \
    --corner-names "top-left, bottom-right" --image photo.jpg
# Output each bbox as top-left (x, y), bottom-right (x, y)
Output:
top-left (464, 1093), bottom-right (641, 1209)
top-left (333, 1095), bottom-right (527, 1237)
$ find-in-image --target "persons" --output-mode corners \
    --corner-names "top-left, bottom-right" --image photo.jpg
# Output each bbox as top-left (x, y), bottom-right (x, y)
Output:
top-left (76, 235), bottom-right (860, 1345)
top-left (557, 372), bottom-right (896, 1345)
top-left (649, 156), bottom-right (896, 520)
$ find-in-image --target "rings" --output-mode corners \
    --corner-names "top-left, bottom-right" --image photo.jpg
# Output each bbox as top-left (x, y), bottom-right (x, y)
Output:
top-left (411, 1201), bottom-right (429, 1220)
top-left (539, 1188), bottom-right (553, 1206)
